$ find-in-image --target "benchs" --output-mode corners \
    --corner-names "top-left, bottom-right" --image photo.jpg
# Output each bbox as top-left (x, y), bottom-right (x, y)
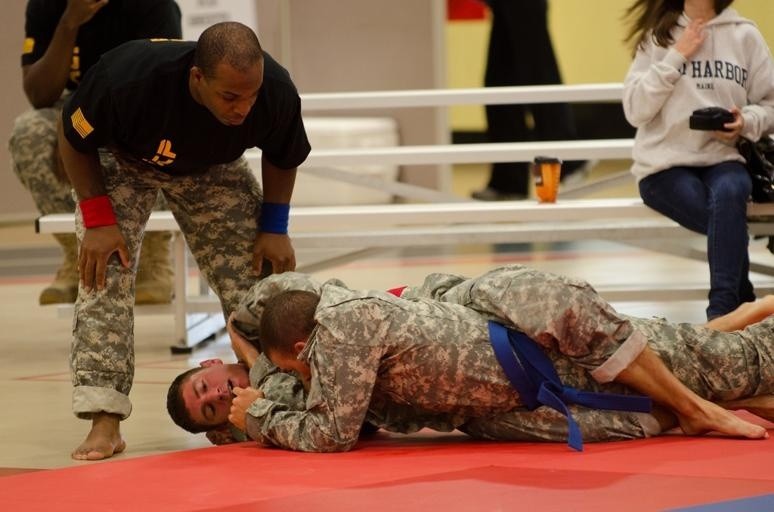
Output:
top-left (33, 78), bottom-right (774, 358)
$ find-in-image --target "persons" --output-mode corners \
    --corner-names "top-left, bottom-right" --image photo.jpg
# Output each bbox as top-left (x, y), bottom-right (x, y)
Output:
top-left (228, 284), bottom-right (774, 443)
top-left (619, 1), bottom-right (774, 326)
top-left (164, 261), bottom-right (772, 440)
top-left (53, 19), bottom-right (310, 458)
top-left (6, 2), bottom-right (185, 308)
top-left (467, 0), bottom-right (591, 204)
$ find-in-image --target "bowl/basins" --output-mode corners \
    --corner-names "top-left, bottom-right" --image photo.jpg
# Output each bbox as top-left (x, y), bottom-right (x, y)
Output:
top-left (688, 105), bottom-right (736, 133)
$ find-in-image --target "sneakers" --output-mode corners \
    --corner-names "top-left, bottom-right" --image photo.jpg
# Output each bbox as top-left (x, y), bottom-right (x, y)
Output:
top-left (470, 187), bottom-right (529, 203)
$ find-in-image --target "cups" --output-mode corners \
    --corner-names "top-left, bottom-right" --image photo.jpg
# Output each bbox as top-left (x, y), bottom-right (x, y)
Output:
top-left (532, 154), bottom-right (564, 205)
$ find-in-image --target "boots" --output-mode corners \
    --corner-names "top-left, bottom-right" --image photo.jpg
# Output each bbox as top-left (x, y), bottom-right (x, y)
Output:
top-left (133, 228), bottom-right (180, 309)
top-left (38, 228), bottom-right (82, 307)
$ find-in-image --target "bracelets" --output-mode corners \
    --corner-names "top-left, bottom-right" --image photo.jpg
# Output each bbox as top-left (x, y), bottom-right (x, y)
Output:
top-left (74, 190), bottom-right (119, 232)
top-left (251, 198), bottom-right (293, 236)
